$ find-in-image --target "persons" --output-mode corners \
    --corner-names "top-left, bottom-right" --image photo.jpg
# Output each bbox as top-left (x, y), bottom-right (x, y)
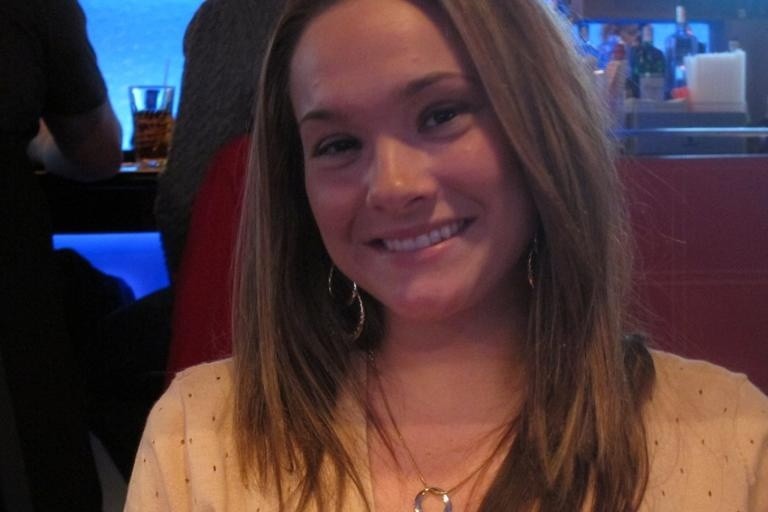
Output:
top-left (121, 0), bottom-right (768, 512)
top-left (81, 0), bottom-right (286, 485)
top-left (0, 0), bottom-right (123, 507)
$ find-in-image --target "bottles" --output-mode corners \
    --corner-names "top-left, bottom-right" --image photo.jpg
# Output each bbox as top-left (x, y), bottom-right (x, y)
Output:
top-left (577, 7), bottom-right (703, 104)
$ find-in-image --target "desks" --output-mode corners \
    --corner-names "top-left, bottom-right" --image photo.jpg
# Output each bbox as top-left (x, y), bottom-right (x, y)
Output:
top-left (3, 144), bottom-right (172, 260)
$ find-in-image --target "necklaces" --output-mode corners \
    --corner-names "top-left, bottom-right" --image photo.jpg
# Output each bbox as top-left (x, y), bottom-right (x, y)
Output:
top-left (368, 345), bottom-right (527, 512)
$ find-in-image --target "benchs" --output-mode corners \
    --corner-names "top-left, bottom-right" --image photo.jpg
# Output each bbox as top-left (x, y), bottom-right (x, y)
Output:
top-left (166, 120), bottom-right (764, 398)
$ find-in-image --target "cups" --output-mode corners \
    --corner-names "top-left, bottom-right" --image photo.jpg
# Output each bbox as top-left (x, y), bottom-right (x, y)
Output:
top-left (126, 85), bottom-right (178, 171)
